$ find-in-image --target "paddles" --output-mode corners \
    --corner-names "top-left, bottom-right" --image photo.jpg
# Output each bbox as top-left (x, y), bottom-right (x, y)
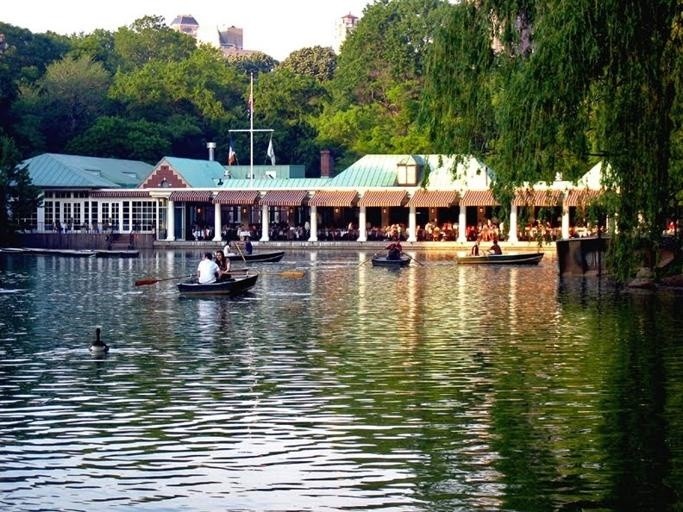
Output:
top-left (216, 270), bottom-right (304, 279)
top-left (235, 241), bottom-right (251, 267)
top-left (135, 268), bottom-right (249, 287)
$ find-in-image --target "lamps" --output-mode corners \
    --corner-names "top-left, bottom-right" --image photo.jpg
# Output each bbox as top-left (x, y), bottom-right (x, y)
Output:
top-left (480, 206), bottom-right (484, 214)
top-left (289, 206), bottom-right (294, 214)
top-left (243, 206), bottom-right (247, 214)
top-left (335, 208), bottom-right (340, 214)
top-left (197, 207), bottom-right (201, 214)
top-left (383, 207), bottom-right (387, 214)
top-left (431, 207), bottom-right (435, 214)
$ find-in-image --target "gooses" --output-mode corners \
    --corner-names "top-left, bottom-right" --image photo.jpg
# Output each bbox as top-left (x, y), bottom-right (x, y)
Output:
top-left (88, 328), bottom-right (108, 351)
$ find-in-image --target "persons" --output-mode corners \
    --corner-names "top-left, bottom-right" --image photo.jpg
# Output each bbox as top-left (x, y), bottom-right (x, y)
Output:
top-left (189, 217), bottom-right (608, 243)
top-left (486, 240), bottom-right (502, 255)
top-left (195, 252), bottom-right (222, 284)
top-left (471, 239), bottom-right (480, 256)
top-left (235, 236), bottom-right (252, 255)
top-left (222, 240), bottom-right (236, 257)
top-left (385, 243), bottom-right (400, 260)
top-left (384, 239), bottom-right (402, 257)
top-left (213, 251), bottom-right (231, 281)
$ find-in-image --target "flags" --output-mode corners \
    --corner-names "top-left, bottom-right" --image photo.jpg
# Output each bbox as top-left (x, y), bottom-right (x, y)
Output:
top-left (246, 93), bottom-right (254, 121)
top-left (226, 135), bottom-right (235, 166)
top-left (266, 139), bottom-right (276, 167)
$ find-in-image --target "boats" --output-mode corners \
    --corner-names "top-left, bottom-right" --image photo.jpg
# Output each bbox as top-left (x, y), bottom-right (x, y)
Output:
top-left (223, 250), bottom-right (285, 263)
top-left (456, 253), bottom-right (544, 265)
top-left (371, 255), bottom-right (410, 267)
top-left (177, 273), bottom-right (257, 297)
